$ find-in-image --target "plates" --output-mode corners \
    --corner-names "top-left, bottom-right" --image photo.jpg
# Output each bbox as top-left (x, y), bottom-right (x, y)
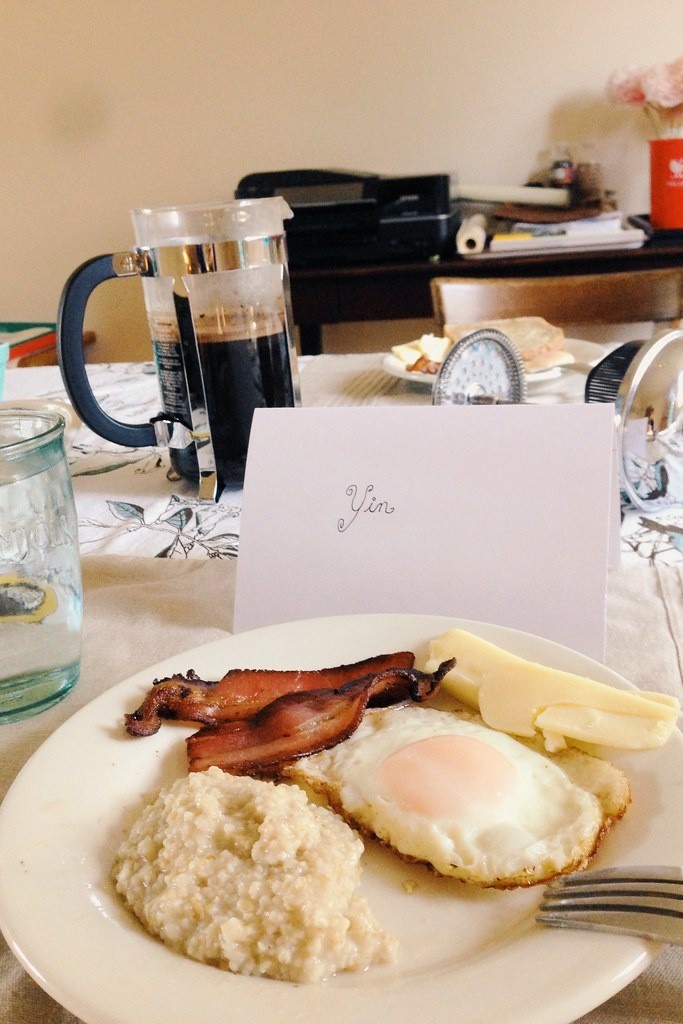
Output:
top-left (0, 613), bottom-right (682, 1024)
top-left (384, 355), bottom-right (561, 391)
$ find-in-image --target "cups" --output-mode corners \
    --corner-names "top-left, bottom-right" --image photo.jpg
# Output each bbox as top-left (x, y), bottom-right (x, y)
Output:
top-left (0, 406), bottom-right (83, 723)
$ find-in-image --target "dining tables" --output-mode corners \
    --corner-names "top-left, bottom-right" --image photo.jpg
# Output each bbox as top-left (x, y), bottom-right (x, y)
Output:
top-left (0, 352), bottom-right (683, 1024)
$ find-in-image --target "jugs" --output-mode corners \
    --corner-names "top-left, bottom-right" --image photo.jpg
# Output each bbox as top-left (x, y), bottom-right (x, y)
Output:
top-left (55, 196), bottom-right (300, 505)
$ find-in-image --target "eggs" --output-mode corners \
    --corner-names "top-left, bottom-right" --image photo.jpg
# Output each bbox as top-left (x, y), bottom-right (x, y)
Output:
top-left (282, 704), bottom-right (604, 889)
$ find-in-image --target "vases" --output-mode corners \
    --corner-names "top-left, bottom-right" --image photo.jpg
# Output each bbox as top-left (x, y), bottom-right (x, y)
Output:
top-left (646, 138), bottom-right (683, 229)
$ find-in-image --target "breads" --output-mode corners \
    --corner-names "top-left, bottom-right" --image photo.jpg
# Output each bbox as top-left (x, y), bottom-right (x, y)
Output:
top-left (442, 315), bottom-right (564, 370)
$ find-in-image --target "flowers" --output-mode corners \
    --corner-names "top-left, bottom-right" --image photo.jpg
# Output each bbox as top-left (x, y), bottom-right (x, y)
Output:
top-left (602, 56), bottom-right (683, 140)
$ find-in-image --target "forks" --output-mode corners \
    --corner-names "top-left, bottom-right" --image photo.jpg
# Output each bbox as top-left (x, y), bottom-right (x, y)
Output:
top-left (536, 864), bottom-right (682, 948)
